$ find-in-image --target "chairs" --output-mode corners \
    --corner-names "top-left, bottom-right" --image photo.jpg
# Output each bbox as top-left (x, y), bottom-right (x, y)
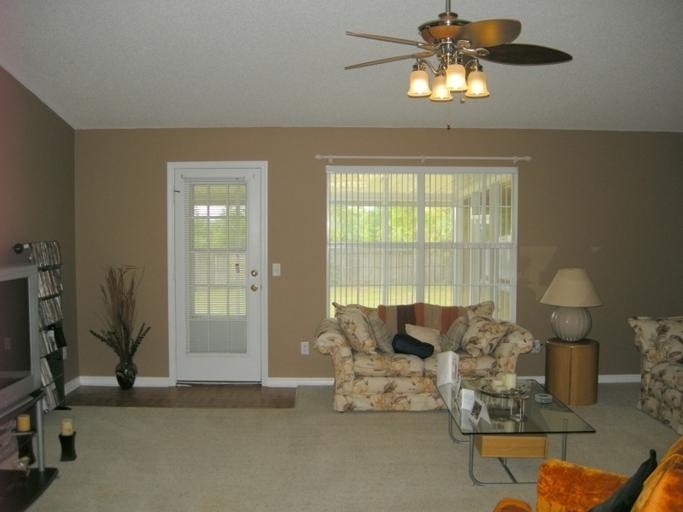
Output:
top-left (627, 314), bottom-right (683, 434)
top-left (492, 437), bottom-right (683, 512)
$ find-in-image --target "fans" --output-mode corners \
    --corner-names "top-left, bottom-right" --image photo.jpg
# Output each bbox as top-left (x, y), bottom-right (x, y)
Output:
top-left (344, 0), bottom-right (572, 70)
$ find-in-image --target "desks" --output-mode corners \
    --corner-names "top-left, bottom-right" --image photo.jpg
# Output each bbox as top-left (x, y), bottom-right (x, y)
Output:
top-left (0, 389), bottom-right (60, 512)
top-left (544, 338), bottom-right (599, 407)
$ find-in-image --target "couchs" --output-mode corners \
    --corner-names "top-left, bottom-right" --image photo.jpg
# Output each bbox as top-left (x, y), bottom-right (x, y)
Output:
top-left (316, 313), bottom-right (535, 411)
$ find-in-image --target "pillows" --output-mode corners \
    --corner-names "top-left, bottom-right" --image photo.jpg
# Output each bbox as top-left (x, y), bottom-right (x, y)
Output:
top-left (331, 301), bottom-right (509, 357)
top-left (585, 449), bottom-right (658, 512)
top-left (655, 318), bottom-right (683, 364)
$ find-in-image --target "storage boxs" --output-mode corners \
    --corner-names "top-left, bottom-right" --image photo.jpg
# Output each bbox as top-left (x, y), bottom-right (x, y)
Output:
top-left (474, 419), bottom-right (549, 457)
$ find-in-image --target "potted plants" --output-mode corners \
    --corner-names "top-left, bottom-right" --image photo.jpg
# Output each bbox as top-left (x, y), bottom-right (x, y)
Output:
top-left (89, 266), bottom-right (151, 389)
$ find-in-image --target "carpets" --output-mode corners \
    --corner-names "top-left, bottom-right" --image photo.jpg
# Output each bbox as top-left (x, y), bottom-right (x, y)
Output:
top-left (0, 381), bottom-right (683, 512)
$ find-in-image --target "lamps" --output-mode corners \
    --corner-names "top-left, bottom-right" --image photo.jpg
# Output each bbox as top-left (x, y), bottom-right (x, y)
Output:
top-left (407, 38), bottom-right (490, 130)
top-left (539, 266), bottom-right (603, 343)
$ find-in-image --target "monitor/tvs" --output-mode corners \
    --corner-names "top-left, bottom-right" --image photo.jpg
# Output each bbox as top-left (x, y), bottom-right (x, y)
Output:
top-left (0, 266), bottom-right (41, 418)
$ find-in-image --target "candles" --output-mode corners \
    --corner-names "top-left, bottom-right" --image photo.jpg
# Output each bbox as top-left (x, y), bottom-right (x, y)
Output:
top-left (61, 417), bottom-right (73, 436)
top-left (17, 414), bottom-right (31, 432)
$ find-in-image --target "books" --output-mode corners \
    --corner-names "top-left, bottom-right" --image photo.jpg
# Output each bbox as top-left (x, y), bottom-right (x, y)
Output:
top-left (28, 239), bottom-right (64, 413)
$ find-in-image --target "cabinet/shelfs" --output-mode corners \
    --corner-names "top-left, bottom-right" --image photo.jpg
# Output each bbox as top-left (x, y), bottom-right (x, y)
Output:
top-left (10, 240), bottom-right (71, 415)
